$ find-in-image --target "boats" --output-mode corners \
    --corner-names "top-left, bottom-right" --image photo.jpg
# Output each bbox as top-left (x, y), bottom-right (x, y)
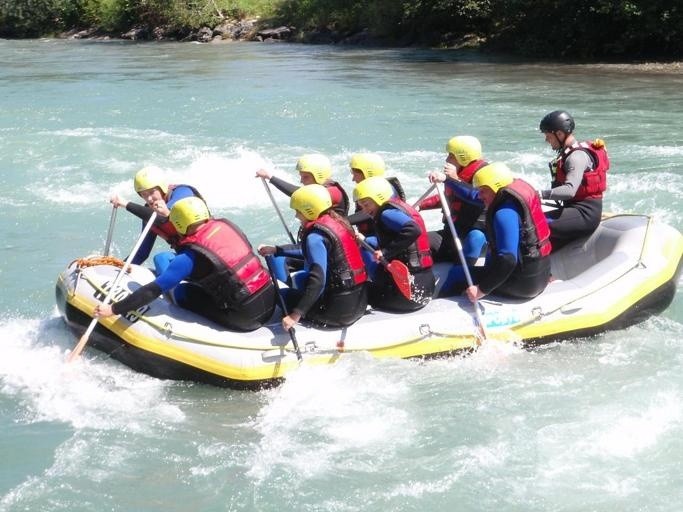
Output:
top-left (56, 214), bottom-right (683, 389)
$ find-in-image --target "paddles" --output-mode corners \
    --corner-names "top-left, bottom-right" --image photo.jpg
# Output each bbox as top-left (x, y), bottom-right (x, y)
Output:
top-left (428, 176), bottom-right (503, 360)
top-left (354, 235), bottom-right (412, 303)
top-left (68, 212), bottom-right (156, 364)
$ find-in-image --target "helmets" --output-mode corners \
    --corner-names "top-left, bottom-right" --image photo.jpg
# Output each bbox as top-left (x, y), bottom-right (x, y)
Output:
top-left (167, 196), bottom-right (210, 236)
top-left (132, 166), bottom-right (169, 196)
top-left (537, 108), bottom-right (577, 134)
top-left (349, 152), bottom-right (386, 178)
top-left (287, 184), bottom-right (335, 222)
top-left (469, 160), bottom-right (514, 193)
top-left (294, 152), bottom-right (332, 184)
top-left (444, 135), bottom-right (483, 168)
top-left (352, 178), bottom-right (394, 207)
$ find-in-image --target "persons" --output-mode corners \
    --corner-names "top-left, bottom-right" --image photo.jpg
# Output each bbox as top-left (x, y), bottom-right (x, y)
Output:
top-left (110, 166), bottom-right (214, 277)
top-left (344, 152), bottom-right (406, 250)
top-left (535, 109), bottom-right (610, 254)
top-left (353, 176), bottom-right (435, 313)
top-left (93, 197), bottom-right (276, 333)
top-left (255, 153), bottom-right (349, 289)
top-left (258, 184), bottom-right (369, 331)
top-left (437, 161), bottom-right (552, 303)
top-left (411, 136), bottom-right (490, 266)
